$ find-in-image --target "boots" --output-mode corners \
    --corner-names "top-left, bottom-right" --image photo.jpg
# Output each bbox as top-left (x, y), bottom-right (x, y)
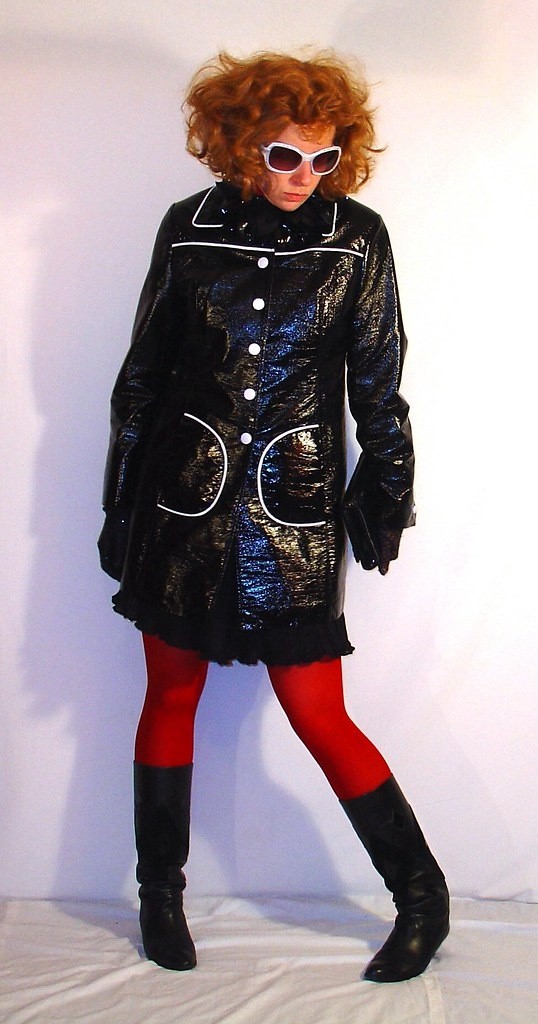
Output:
top-left (133, 761), bottom-right (196, 971)
top-left (339, 775), bottom-right (449, 982)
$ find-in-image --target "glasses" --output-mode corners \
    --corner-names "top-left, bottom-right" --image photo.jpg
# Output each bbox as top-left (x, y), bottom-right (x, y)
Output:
top-left (260, 142), bottom-right (342, 175)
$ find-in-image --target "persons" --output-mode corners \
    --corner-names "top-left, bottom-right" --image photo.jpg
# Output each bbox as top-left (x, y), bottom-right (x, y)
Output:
top-left (97, 49), bottom-right (450, 986)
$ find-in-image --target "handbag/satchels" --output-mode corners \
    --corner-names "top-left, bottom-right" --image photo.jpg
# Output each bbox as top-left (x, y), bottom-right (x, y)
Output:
top-left (343, 504), bottom-right (379, 571)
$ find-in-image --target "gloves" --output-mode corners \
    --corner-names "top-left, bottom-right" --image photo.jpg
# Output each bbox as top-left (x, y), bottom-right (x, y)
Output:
top-left (352, 527), bottom-right (402, 575)
top-left (97, 518), bottom-right (126, 582)
